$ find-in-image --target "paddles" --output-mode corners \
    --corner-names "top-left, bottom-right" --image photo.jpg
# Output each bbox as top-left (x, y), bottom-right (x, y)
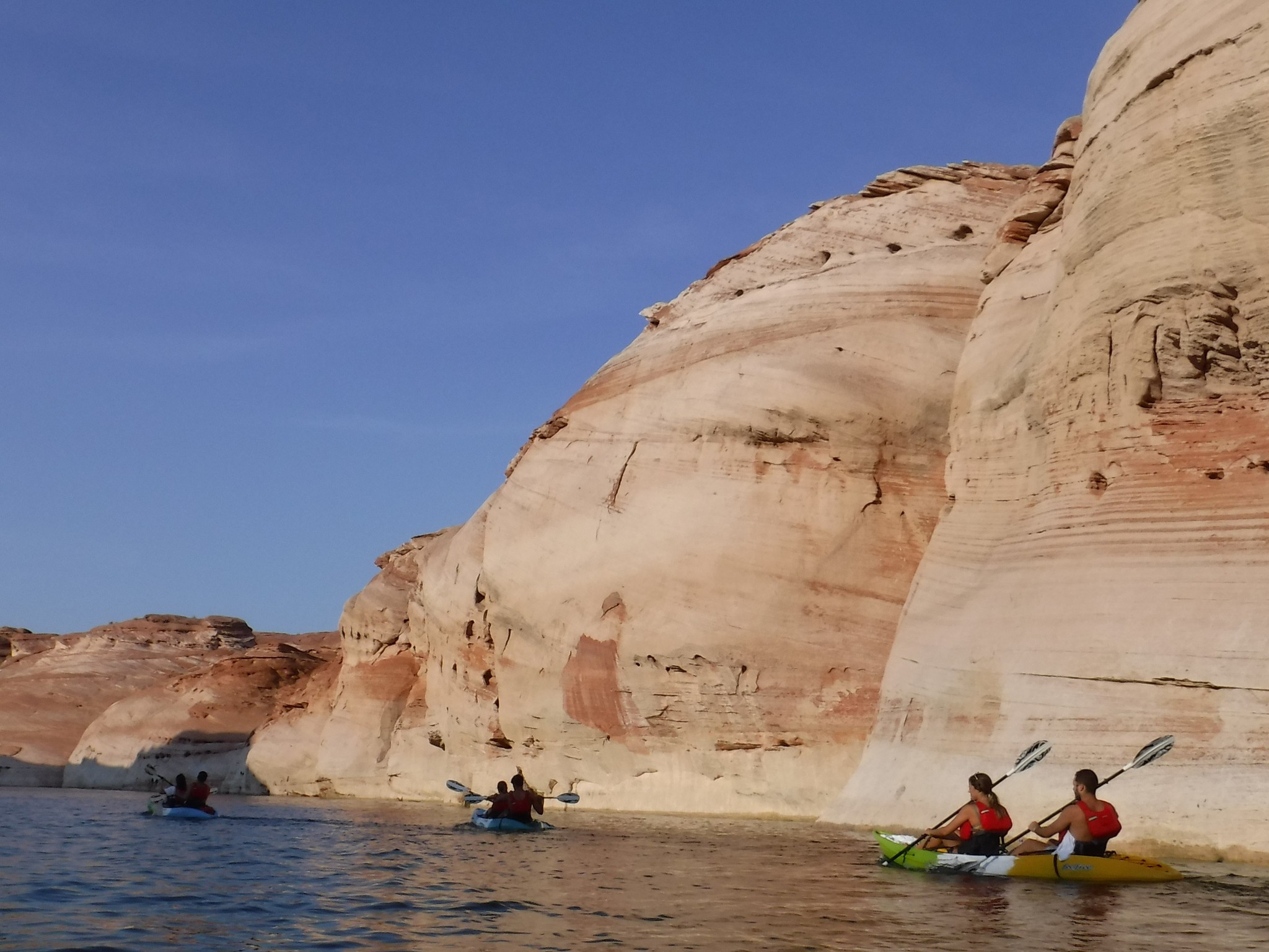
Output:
top-left (462, 792), bottom-right (580, 805)
top-left (928, 734), bottom-right (1176, 885)
top-left (143, 762), bottom-right (176, 785)
top-left (876, 737), bottom-right (1055, 876)
top-left (445, 780), bottom-right (557, 829)
top-left (149, 787), bottom-right (219, 803)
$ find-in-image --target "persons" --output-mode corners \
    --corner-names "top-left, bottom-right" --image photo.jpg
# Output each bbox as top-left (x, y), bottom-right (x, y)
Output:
top-left (479, 774), bottom-right (544, 824)
top-left (158, 771), bottom-right (210, 810)
top-left (905, 772), bottom-right (1000, 858)
top-left (1011, 769), bottom-right (1119, 857)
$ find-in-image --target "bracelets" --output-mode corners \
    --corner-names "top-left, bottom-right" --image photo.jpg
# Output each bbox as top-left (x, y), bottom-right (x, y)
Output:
top-left (1033, 826), bottom-right (1041, 832)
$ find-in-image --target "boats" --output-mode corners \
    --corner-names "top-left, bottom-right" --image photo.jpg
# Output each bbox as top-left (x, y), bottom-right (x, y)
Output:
top-left (471, 808), bottom-right (541, 831)
top-left (872, 829), bottom-right (1183, 882)
top-left (148, 794), bottom-right (219, 819)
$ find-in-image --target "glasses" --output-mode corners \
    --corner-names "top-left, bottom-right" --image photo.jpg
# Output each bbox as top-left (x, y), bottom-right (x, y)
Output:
top-left (974, 774), bottom-right (978, 780)
